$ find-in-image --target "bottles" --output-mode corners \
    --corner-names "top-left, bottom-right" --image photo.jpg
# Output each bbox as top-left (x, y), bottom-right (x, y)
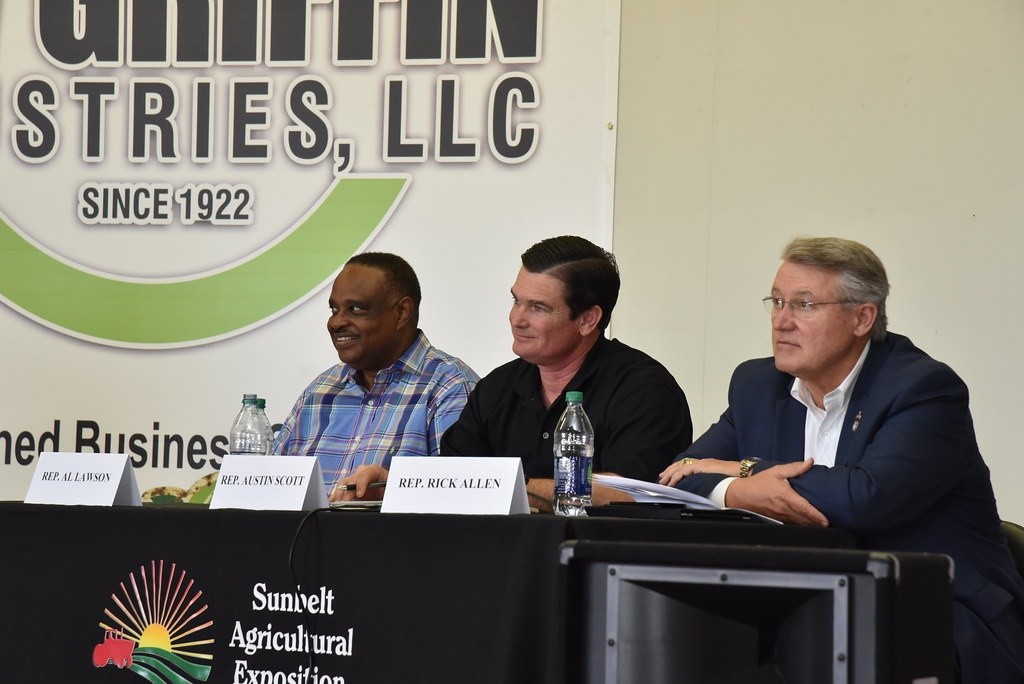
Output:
top-left (553, 391), bottom-right (592, 518)
top-left (230, 394), bottom-right (267, 457)
top-left (256, 399), bottom-right (275, 456)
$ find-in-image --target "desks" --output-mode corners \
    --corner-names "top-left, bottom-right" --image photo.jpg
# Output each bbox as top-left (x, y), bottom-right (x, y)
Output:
top-left (0, 501), bottom-right (859, 684)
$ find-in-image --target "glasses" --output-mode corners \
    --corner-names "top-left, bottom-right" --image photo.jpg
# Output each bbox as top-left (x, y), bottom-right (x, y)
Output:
top-left (763, 296), bottom-right (858, 318)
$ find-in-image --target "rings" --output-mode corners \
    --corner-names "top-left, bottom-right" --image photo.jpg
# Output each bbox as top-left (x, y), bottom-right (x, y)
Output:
top-left (683, 458), bottom-right (693, 467)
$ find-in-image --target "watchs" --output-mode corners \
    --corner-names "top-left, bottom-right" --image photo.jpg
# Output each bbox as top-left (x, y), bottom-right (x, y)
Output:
top-left (740, 456), bottom-right (761, 479)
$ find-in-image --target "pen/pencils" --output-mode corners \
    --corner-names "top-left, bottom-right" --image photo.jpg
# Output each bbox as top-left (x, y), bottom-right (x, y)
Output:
top-left (336, 481), bottom-right (387, 491)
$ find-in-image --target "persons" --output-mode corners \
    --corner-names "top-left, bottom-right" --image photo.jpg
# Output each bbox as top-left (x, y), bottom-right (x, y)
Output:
top-left (330, 235), bottom-right (692, 513)
top-left (659, 239), bottom-right (1007, 684)
top-left (243, 253), bottom-right (480, 509)
